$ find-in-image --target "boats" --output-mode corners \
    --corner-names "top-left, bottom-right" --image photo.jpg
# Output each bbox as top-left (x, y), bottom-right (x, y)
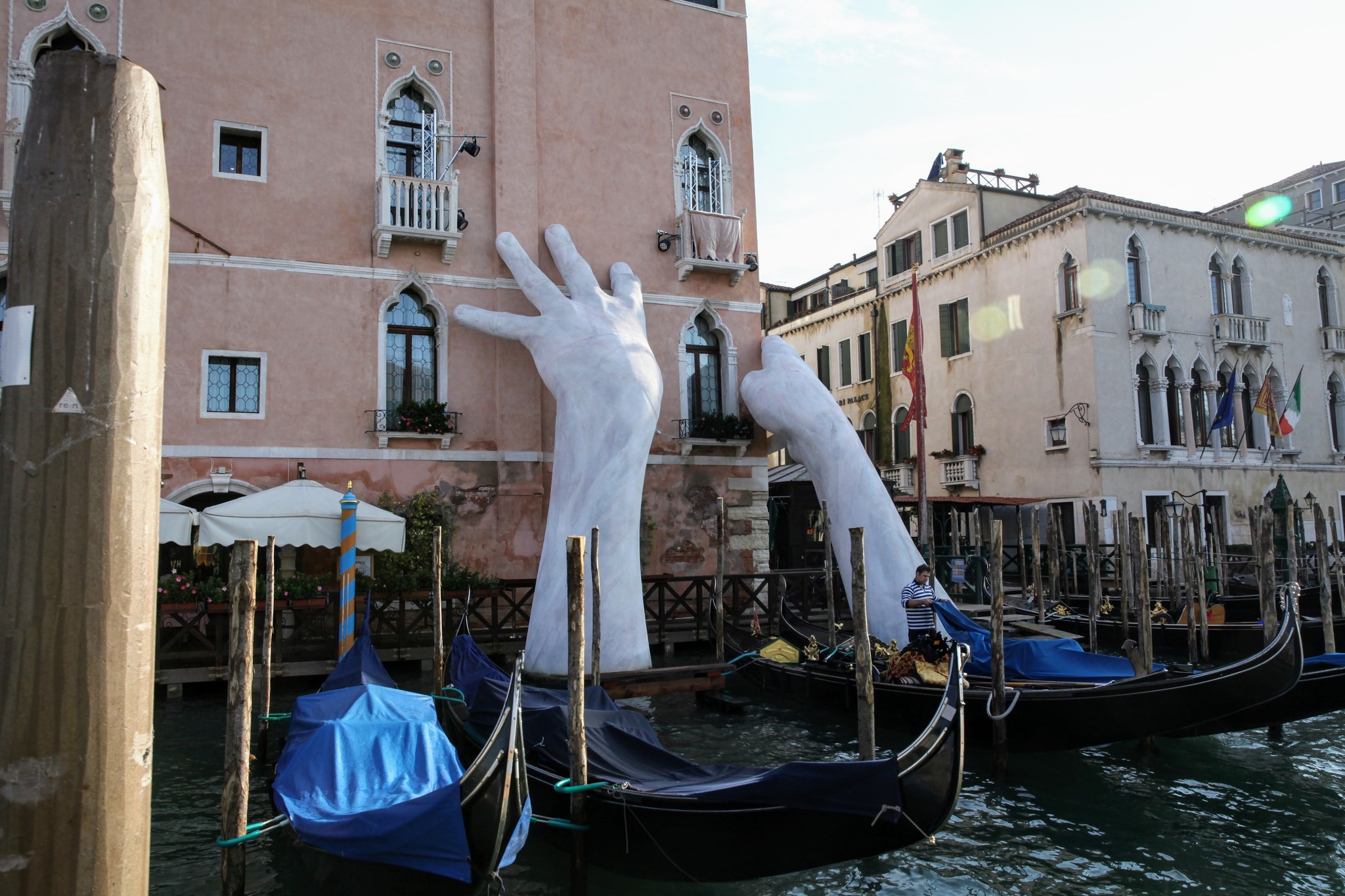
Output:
top-left (267, 583), bottom-right (474, 888)
top-left (440, 579), bottom-right (971, 886)
top-left (778, 565), bottom-right (1344, 746)
top-left (703, 563), bottom-right (1306, 757)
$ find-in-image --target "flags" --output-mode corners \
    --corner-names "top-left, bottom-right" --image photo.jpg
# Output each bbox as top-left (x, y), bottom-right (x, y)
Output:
top-left (1210, 366), bottom-right (1235, 430)
top-left (1252, 375), bottom-right (1285, 442)
top-left (1278, 369), bottom-right (1302, 435)
top-left (899, 269), bottom-right (931, 430)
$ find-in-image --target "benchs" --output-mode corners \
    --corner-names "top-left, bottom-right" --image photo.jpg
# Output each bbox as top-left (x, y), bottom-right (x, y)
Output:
top-left (1176, 601), bottom-right (1225, 625)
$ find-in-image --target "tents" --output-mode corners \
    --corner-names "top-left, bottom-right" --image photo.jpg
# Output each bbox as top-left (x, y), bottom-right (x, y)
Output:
top-left (160, 496), bottom-right (201, 548)
top-left (194, 478), bottom-right (412, 624)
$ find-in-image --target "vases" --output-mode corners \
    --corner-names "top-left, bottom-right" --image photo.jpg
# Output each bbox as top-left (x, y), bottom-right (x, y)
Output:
top-left (944, 452), bottom-right (955, 457)
top-left (934, 454), bottom-right (943, 459)
top-left (158, 597), bottom-right (326, 614)
top-left (969, 449), bottom-right (982, 454)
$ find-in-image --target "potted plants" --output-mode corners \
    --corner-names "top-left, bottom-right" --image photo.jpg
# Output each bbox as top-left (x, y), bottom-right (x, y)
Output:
top-left (356, 559), bottom-right (504, 604)
top-left (875, 455), bottom-right (917, 467)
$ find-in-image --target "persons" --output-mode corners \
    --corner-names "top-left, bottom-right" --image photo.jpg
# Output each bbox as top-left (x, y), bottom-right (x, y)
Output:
top-left (454, 222), bottom-right (665, 674)
top-left (901, 565), bottom-right (951, 641)
top-left (740, 334), bottom-right (959, 649)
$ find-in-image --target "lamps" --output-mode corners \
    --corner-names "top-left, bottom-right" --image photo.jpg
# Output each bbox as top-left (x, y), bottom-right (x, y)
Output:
top-left (457, 210), bottom-right (468, 231)
top-left (658, 234), bottom-right (671, 252)
top-left (465, 136), bottom-right (480, 157)
top-left (745, 254), bottom-right (758, 272)
top-left (716, 437), bottom-right (727, 442)
top-left (1049, 403), bottom-right (1090, 444)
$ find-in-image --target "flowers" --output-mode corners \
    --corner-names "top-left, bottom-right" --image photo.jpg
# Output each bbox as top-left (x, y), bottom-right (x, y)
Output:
top-left (156, 566), bottom-right (334, 606)
top-left (395, 396), bottom-right (455, 436)
top-left (691, 410), bottom-right (751, 439)
top-left (928, 448), bottom-right (952, 460)
top-left (970, 445), bottom-right (986, 451)
